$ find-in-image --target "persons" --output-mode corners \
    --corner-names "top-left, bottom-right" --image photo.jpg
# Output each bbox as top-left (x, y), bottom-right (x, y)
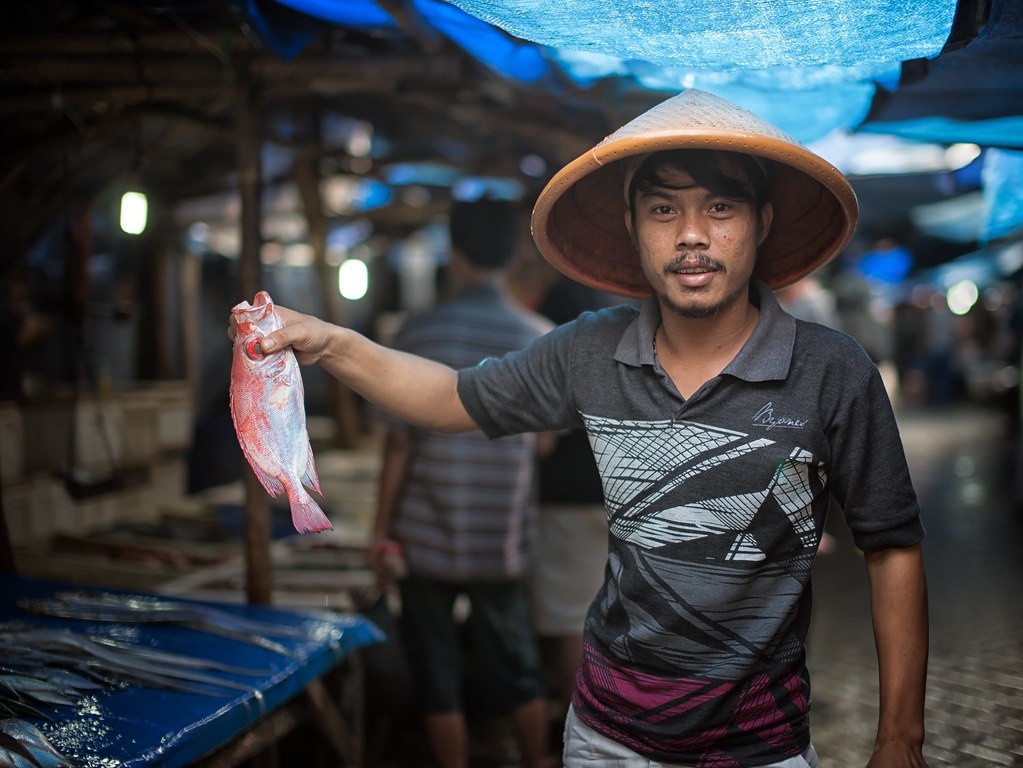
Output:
top-left (228, 88), bottom-right (929, 768)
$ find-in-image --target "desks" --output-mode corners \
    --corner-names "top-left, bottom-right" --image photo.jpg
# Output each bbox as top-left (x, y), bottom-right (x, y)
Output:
top-left (0, 569), bottom-right (388, 768)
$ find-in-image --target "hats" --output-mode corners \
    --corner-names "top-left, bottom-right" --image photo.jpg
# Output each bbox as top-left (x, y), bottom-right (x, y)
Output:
top-left (530, 88), bottom-right (859, 299)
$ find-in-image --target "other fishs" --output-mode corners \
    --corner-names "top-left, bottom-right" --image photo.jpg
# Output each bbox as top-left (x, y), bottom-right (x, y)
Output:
top-left (0, 589), bottom-right (296, 768)
top-left (230, 290), bottom-right (334, 535)
